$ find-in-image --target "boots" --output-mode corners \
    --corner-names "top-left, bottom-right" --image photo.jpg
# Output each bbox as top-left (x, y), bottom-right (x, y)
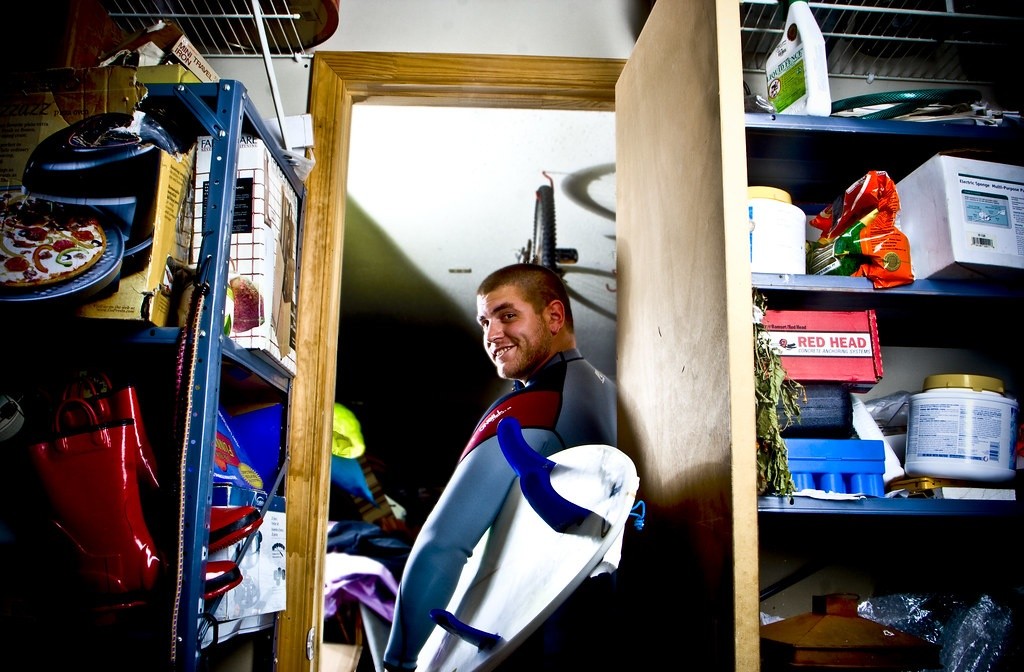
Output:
top-left (47, 370), bottom-right (263, 557)
top-left (27, 397), bottom-right (242, 627)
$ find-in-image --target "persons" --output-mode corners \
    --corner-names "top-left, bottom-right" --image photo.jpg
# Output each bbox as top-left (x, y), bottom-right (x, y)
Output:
top-left (380, 264), bottom-right (617, 672)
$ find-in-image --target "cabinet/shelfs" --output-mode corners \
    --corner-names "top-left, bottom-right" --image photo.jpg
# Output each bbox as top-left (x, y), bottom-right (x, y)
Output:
top-left (0, 77), bottom-right (307, 672)
top-left (613, 0), bottom-right (1024, 672)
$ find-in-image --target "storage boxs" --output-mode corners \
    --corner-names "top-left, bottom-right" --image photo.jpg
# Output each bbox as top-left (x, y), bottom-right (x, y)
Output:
top-left (0, 92), bottom-right (190, 328)
top-left (763, 307), bottom-right (883, 394)
top-left (205, 483), bottom-right (287, 623)
top-left (193, 132), bottom-right (299, 379)
top-left (894, 146), bottom-right (1024, 284)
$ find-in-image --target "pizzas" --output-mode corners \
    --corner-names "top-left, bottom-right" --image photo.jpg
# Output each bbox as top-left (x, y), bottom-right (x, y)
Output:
top-left (0, 199), bottom-right (107, 290)
